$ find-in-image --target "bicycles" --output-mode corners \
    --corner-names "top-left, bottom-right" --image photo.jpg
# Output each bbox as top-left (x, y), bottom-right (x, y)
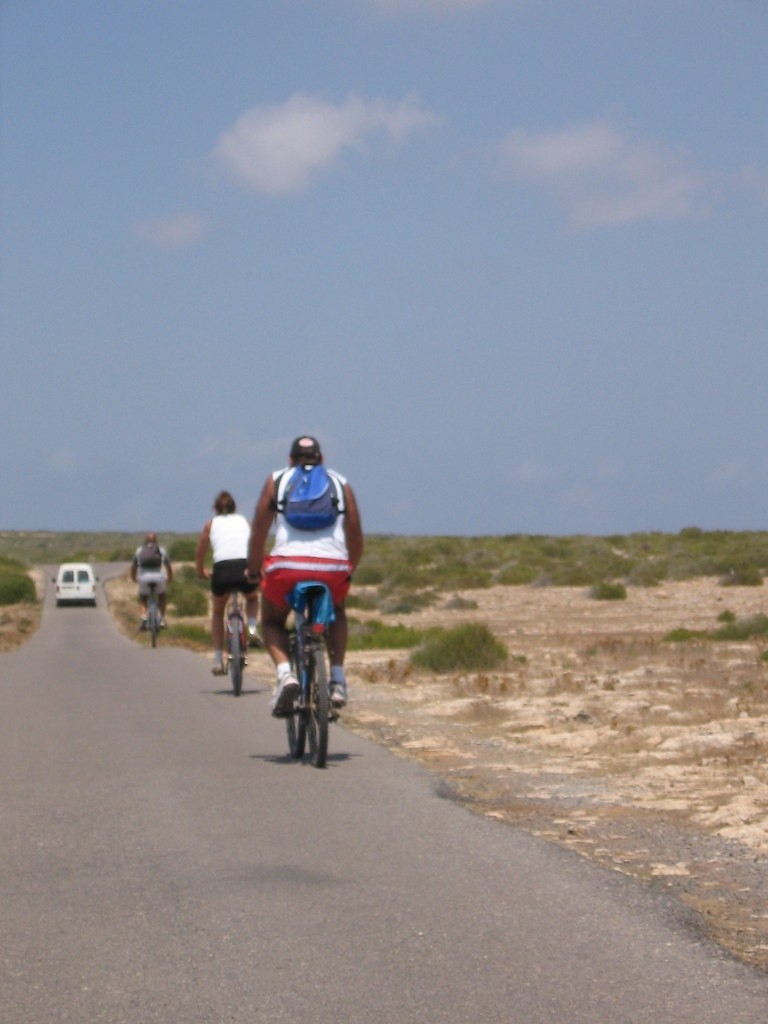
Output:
top-left (199, 573), bottom-right (260, 696)
top-left (249, 574), bottom-right (343, 767)
top-left (141, 582), bottom-right (163, 648)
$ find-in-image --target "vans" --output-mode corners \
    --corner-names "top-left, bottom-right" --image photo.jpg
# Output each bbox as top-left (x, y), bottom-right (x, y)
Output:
top-left (52, 562), bottom-right (99, 607)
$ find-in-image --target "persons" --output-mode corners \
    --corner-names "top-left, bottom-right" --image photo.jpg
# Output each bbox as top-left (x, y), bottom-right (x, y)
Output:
top-left (130, 532), bottom-right (172, 633)
top-left (196, 491), bottom-right (261, 676)
top-left (244, 436), bottom-right (364, 719)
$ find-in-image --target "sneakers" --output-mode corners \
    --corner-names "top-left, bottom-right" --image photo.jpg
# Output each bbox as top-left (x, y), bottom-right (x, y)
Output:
top-left (327, 682), bottom-right (347, 722)
top-left (271, 673), bottom-right (301, 718)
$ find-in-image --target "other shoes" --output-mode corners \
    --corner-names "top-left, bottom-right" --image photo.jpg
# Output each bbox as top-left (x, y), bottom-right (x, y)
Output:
top-left (140, 618), bottom-right (148, 631)
top-left (213, 666), bottom-right (223, 675)
top-left (248, 632), bottom-right (258, 647)
top-left (160, 620), bottom-right (165, 628)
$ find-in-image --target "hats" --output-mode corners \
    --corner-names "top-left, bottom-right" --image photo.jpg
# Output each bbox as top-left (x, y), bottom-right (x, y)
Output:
top-left (291, 437), bottom-right (319, 456)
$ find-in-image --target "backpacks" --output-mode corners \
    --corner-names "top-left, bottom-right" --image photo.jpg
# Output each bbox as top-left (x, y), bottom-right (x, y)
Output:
top-left (138, 542), bottom-right (162, 567)
top-left (275, 462), bottom-right (345, 529)
